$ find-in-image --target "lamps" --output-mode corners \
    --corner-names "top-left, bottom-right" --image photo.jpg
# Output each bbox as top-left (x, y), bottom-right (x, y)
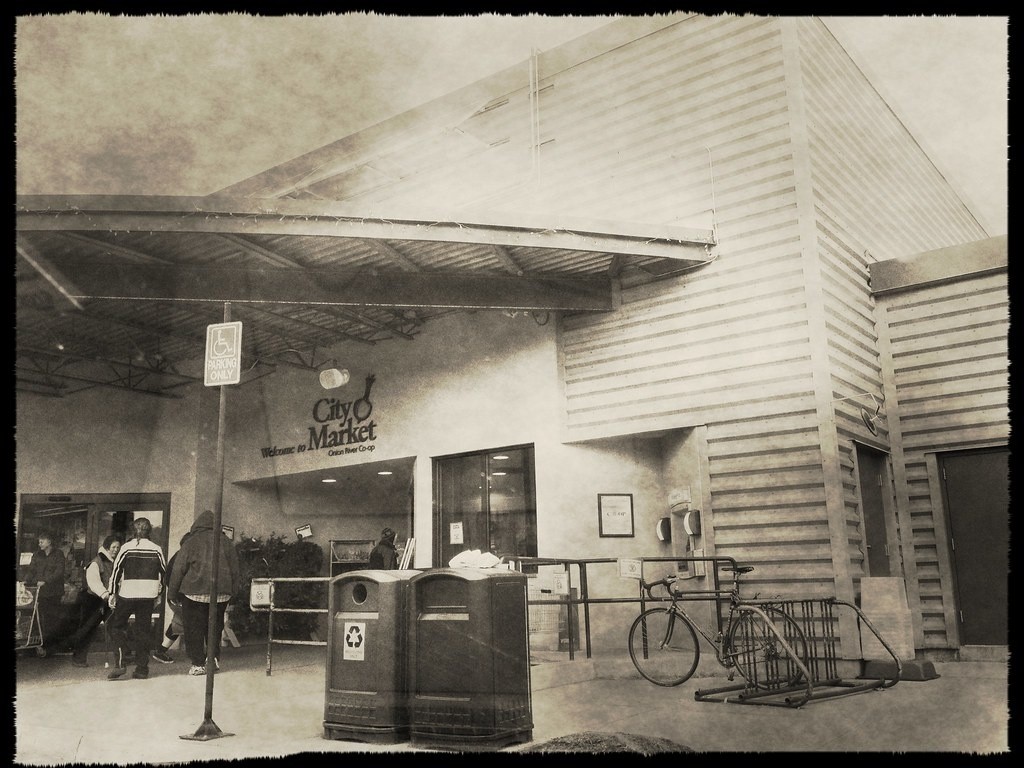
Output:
top-left (319, 360), bottom-right (350, 389)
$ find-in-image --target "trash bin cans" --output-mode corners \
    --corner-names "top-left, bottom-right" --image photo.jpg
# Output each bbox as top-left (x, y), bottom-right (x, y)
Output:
top-left (323, 568), bottom-right (424, 745)
top-left (405, 568), bottom-right (534, 753)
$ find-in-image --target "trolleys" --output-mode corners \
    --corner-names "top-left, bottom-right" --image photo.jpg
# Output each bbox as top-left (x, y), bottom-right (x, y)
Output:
top-left (15, 583), bottom-right (45, 659)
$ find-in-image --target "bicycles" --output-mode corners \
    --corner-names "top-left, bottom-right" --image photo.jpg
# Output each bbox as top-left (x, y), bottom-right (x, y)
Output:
top-left (628, 565), bottom-right (809, 686)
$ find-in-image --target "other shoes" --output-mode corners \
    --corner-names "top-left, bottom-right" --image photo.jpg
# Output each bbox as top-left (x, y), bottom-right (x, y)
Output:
top-left (108, 666), bottom-right (126, 678)
top-left (72, 661), bottom-right (89, 668)
top-left (134, 666), bottom-right (149, 678)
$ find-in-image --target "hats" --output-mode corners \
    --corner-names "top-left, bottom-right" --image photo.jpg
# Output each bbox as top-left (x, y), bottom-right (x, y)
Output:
top-left (381, 527), bottom-right (395, 545)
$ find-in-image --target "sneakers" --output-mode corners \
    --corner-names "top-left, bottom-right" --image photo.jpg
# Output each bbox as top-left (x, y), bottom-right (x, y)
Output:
top-left (153, 650), bottom-right (175, 663)
top-left (205, 657), bottom-right (219, 669)
top-left (189, 665), bottom-right (205, 674)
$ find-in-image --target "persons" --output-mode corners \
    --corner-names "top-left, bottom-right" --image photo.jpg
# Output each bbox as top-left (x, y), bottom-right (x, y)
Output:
top-left (367, 526), bottom-right (398, 570)
top-left (27, 510), bottom-right (241, 678)
